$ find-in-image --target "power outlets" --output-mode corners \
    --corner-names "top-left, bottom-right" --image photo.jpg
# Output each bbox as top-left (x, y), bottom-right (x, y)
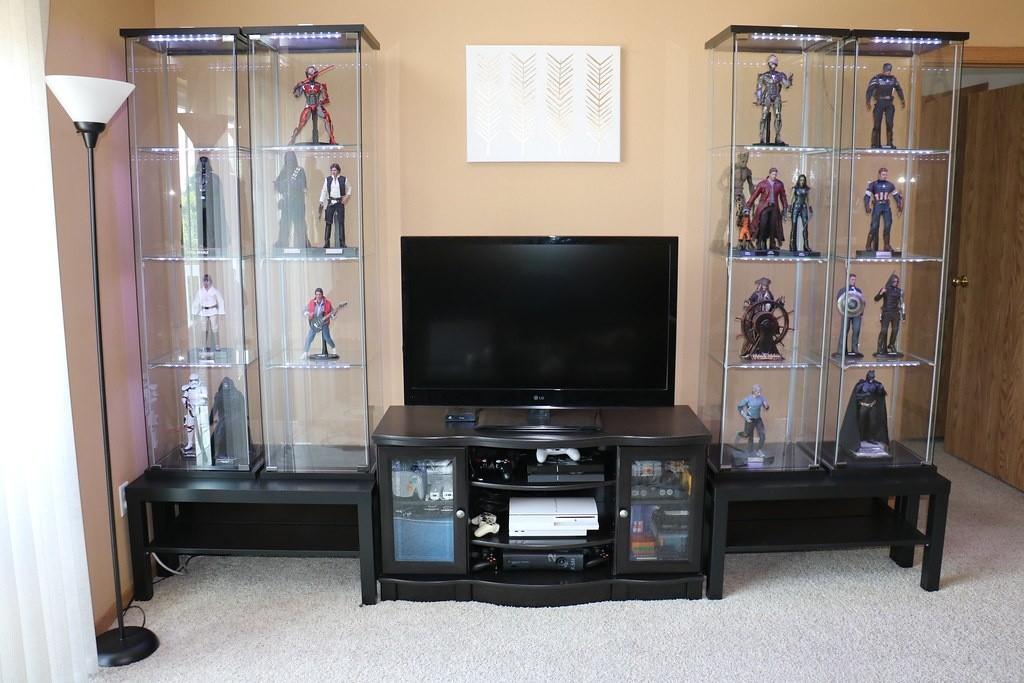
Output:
top-left (118, 481), bottom-right (128, 518)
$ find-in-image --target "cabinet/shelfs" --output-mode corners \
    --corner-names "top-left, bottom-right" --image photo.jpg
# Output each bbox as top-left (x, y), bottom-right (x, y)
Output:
top-left (238, 26), bottom-right (383, 490)
top-left (374, 407), bottom-right (711, 608)
top-left (119, 24), bottom-right (282, 482)
top-left (703, 23), bottom-right (852, 475)
top-left (797, 28), bottom-right (971, 477)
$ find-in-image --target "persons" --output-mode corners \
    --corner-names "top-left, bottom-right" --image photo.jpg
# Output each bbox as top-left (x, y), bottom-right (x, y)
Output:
top-left (839, 369), bottom-right (890, 452)
top-left (181, 156), bottom-right (232, 248)
top-left (874, 274), bottom-right (906, 354)
top-left (299, 288), bottom-right (338, 360)
top-left (740, 277), bottom-right (785, 356)
top-left (191, 274), bottom-right (226, 351)
top-left (837, 273), bottom-right (864, 355)
top-left (788, 174), bottom-right (813, 252)
top-left (181, 373), bottom-right (208, 452)
top-left (755, 55), bottom-right (793, 144)
top-left (737, 384), bottom-right (769, 458)
top-left (863, 168), bottom-right (902, 252)
top-left (287, 66), bottom-right (335, 147)
top-left (319, 163), bottom-right (352, 248)
top-left (746, 167), bottom-right (788, 251)
top-left (209, 376), bottom-right (256, 458)
top-left (866, 63), bottom-right (905, 149)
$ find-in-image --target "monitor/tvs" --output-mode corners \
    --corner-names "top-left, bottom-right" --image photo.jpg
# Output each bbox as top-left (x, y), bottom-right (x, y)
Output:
top-left (401, 235), bottom-right (678, 433)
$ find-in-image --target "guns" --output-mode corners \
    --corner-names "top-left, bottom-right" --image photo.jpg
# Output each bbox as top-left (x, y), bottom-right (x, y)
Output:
top-left (318, 204), bottom-right (323, 219)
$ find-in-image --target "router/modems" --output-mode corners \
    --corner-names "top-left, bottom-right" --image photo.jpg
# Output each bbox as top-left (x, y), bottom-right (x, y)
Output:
top-left (446, 408), bottom-right (479, 421)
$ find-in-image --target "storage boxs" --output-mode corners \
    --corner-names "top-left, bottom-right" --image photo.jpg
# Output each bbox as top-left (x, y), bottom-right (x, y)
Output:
top-left (392, 516), bottom-right (455, 563)
top-left (631, 461), bottom-right (662, 478)
top-left (663, 510), bottom-right (690, 531)
top-left (632, 504), bottom-right (659, 536)
top-left (650, 521), bottom-right (689, 560)
top-left (631, 533), bottom-right (658, 560)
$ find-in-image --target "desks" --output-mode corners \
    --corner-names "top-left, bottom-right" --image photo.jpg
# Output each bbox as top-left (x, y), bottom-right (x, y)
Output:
top-left (124, 446), bottom-right (380, 608)
top-left (707, 440), bottom-right (954, 605)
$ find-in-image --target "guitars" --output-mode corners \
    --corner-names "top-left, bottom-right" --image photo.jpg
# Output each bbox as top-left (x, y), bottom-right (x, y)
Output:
top-left (309, 302), bottom-right (348, 333)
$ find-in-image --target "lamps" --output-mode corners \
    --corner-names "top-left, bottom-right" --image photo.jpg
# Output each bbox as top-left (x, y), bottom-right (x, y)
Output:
top-left (45, 74), bottom-right (159, 668)
